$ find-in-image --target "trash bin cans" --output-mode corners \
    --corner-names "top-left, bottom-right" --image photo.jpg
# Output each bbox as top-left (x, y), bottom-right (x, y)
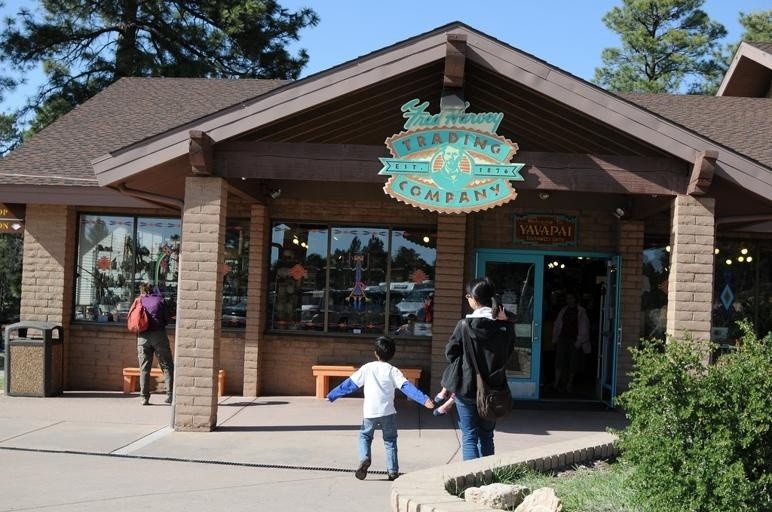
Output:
top-left (4, 320), bottom-right (64, 398)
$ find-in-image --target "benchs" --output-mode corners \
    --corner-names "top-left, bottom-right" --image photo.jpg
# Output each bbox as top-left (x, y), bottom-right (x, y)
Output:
top-left (122, 367), bottom-right (225, 396)
top-left (312, 365), bottom-right (423, 402)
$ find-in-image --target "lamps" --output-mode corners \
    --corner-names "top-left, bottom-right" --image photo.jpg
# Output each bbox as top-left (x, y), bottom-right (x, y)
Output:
top-left (611, 206), bottom-right (630, 220)
top-left (264, 183), bottom-right (282, 200)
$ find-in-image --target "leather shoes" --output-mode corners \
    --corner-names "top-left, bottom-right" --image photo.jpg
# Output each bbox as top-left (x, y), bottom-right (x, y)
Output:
top-left (433, 406), bottom-right (446, 416)
top-left (434, 393), bottom-right (449, 403)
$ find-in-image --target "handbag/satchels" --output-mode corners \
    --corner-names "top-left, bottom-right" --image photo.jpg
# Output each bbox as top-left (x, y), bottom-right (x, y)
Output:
top-left (476, 387), bottom-right (512, 419)
top-left (126, 300), bottom-right (147, 332)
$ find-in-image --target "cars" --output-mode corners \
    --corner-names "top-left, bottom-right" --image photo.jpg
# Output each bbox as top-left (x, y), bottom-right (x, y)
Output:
top-left (296, 280), bottom-right (435, 322)
top-left (109, 302), bottom-right (131, 322)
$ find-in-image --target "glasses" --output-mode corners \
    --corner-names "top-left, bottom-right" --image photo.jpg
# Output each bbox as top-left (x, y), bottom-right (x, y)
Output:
top-left (464, 295), bottom-right (472, 298)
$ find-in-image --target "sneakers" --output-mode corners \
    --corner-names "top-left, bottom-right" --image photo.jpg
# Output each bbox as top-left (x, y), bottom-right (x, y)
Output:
top-left (354, 460), bottom-right (372, 480)
top-left (140, 397), bottom-right (172, 405)
top-left (388, 472), bottom-right (398, 482)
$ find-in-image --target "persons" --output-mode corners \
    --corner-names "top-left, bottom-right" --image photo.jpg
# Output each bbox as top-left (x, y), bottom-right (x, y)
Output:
top-left (439, 276), bottom-right (512, 461)
top-left (432, 306), bottom-right (516, 415)
top-left (551, 290), bottom-right (590, 394)
top-left (325, 335), bottom-right (435, 480)
top-left (134, 282), bottom-right (176, 405)
top-left (396, 296), bottom-right (435, 335)
